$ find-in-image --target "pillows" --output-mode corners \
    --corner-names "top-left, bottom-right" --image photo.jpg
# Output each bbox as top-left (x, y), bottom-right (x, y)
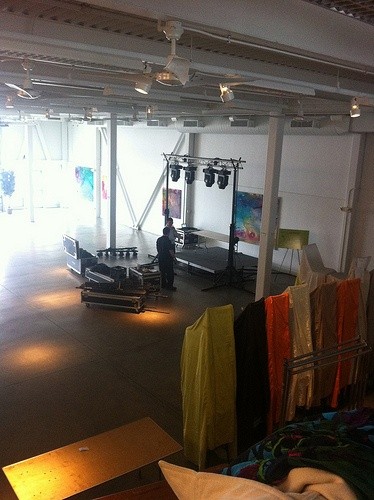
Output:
top-left (156, 459), bottom-right (295, 500)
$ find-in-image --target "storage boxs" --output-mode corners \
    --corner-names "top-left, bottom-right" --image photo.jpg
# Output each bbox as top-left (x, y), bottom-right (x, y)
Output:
top-left (62, 234), bottom-right (162, 314)
top-left (175, 227), bottom-right (200, 245)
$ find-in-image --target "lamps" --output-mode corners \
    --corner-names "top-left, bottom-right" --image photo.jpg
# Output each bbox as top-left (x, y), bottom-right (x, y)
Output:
top-left (219, 82), bottom-right (235, 103)
top-left (133, 63), bottom-right (154, 96)
top-left (350, 97), bottom-right (361, 118)
top-left (169, 161), bottom-right (232, 190)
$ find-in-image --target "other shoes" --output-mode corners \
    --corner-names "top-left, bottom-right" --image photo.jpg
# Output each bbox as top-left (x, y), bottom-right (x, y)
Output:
top-left (161, 285), bottom-right (167, 288)
top-left (167, 287), bottom-right (176, 291)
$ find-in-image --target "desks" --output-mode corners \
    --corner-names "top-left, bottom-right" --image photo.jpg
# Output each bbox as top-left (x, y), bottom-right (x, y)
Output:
top-left (2, 416), bottom-right (185, 500)
top-left (192, 230), bottom-right (242, 263)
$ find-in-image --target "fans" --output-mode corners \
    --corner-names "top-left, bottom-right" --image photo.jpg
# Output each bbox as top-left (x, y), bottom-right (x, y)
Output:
top-left (0, 60), bottom-right (97, 101)
top-left (91, 20), bottom-right (263, 89)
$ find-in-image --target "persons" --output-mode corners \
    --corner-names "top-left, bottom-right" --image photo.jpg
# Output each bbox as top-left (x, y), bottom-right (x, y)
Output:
top-left (156, 227), bottom-right (177, 290)
top-left (166, 218), bottom-right (177, 254)
